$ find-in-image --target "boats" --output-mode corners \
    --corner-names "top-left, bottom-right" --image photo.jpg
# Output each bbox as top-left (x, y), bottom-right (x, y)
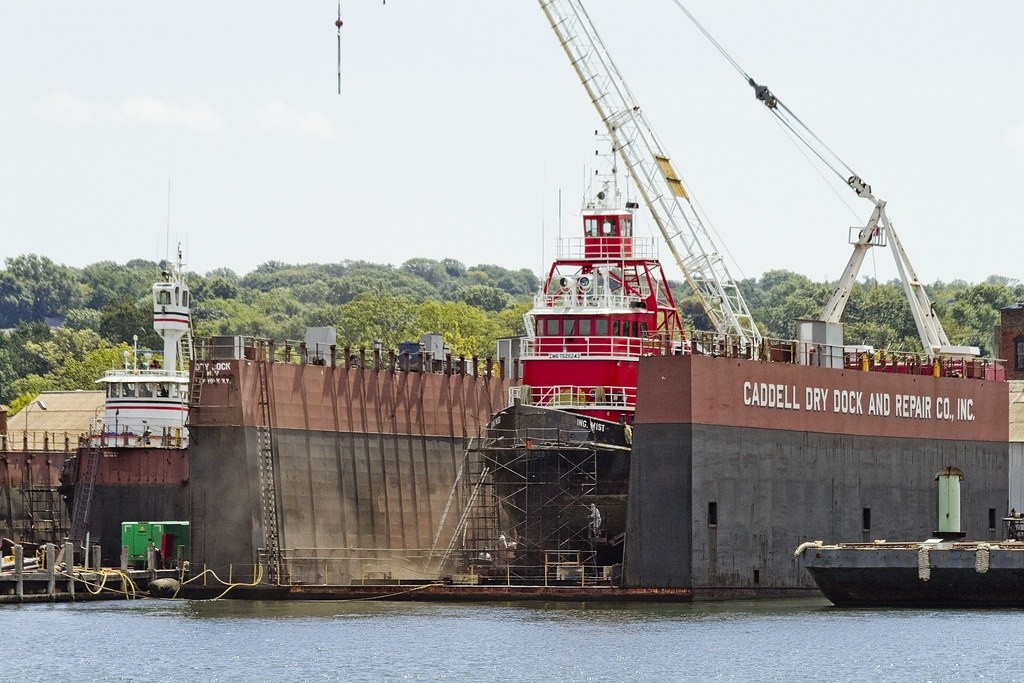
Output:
top-left (461, 117), bottom-right (1005, 582)
top-left (37, 176), bottom-right (208, 570)
top-left (794, 507), bottom-right (1023, 609)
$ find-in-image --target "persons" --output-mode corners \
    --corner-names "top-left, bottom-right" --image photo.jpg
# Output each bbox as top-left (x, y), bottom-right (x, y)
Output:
top-left (624, 423), bottom-right (632, 444)
top-left (479, 552), bottom-right (492, 560)
top-left (588, 503), bottom-right (601, 538)
top-left (145, 426), bottom-right (151, 436)
top-left (499, 531), bottom-right (508, 549)
